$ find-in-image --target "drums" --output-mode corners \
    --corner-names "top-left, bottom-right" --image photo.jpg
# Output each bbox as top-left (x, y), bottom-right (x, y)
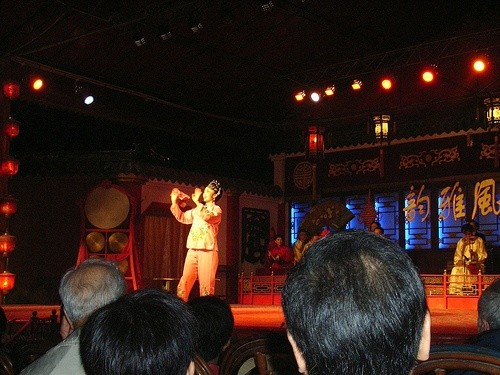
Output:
top-left (83, 182), bottom-right (131, 273)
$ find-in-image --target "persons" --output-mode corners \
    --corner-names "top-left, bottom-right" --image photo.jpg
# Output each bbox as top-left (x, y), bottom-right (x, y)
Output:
top-left (170, 179), bottom-right (223, 304)
top-left (449, 222), bottom-right (487, 295)
top-left (20, 222), bottom-right (430, 375)
top-left (445, 277), bottom-right (500, 375)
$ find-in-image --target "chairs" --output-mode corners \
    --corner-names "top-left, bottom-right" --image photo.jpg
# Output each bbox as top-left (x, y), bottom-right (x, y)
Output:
top-left (0, 333), bottom-right (500, 375)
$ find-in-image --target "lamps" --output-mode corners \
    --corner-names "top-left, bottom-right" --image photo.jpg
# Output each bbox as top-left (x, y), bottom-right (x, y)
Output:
top-left (373, 114), bottom-right (391, 139)
top-left (484, 97), bottom-right (500, 126)
top-left (305, 126), bottom-right (325, 160)
